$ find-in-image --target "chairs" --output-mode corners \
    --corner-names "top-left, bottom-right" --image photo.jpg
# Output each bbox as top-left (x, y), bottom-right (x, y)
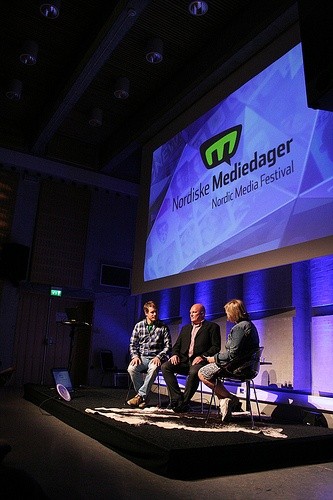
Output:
top-left (123, 339), bottom-right (265, 429)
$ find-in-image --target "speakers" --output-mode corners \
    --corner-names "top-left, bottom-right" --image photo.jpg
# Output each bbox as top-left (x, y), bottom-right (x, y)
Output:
top-left (270, 406), bottom-right (328, 427)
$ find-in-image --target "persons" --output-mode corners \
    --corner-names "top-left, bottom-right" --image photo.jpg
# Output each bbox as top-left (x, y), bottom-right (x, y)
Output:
top-left (161, 303), bottom-right (221, 412)
top-left (198, 299), bottom-right (260, 422)
top-left (126, 301), bottom-right (172, 408)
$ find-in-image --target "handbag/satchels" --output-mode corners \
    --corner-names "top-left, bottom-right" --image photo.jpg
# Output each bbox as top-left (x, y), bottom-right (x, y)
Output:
top-left (167, 400), bottom-right (178, 409)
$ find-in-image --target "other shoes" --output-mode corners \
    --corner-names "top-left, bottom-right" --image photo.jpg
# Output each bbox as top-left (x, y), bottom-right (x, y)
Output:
top-left (127, 395), bottom-right (142, 407)
top-left (139, 400), bottom-right (148, 408)
top-left (173, 402), bottom-right (191, 413)
top-left (219, 398), bottom-right (232, 422)
top-left (231, 395), bottom-right (239, 409)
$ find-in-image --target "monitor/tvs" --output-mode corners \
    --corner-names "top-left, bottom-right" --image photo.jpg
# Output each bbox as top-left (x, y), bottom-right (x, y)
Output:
top-left (50, 367), bottom-right (74, 392)
top-left (99, 264), bottom-right (132, 289)
